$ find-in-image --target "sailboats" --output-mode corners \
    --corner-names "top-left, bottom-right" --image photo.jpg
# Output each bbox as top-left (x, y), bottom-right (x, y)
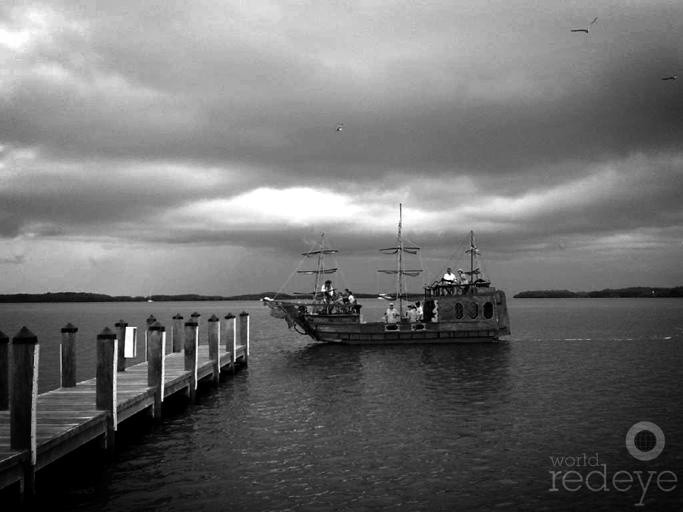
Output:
top-left (261, 203), bottom-right (511, 346)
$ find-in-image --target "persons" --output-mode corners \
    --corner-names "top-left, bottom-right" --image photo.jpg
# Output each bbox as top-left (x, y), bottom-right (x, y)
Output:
top-left (320, 278), bottom-right (335, 305)
top-left (404, 300), bottom-right (424, 325)
top-left (330, 288), bottom-right (356, 315)
top-left (440, 266), bottom-right (458, 285)
top-left (455, 268), bottom-right (466, 285)
top-left (382, 302), bottom-right (401, 323)
